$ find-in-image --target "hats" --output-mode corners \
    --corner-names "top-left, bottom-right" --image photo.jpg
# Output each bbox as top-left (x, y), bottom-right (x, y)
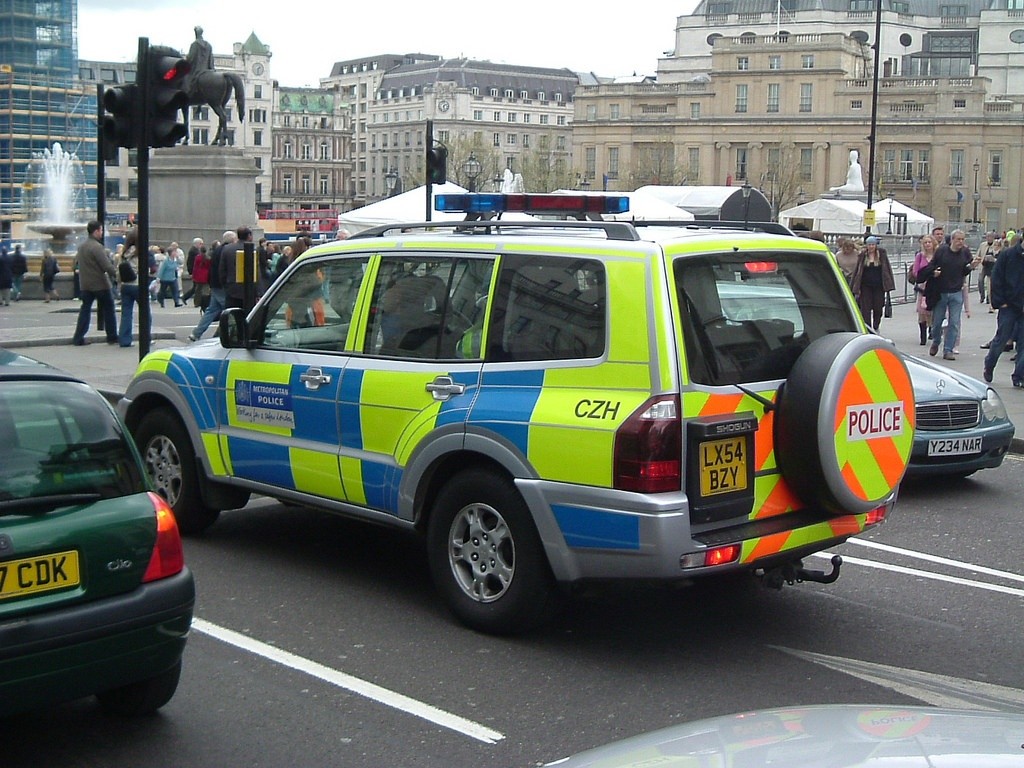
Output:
top-left (866, 236), bottom-right (877, 244)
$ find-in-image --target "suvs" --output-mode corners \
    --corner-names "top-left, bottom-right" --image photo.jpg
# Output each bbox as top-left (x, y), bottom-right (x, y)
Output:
top-left (112, 191), bottom-right (915, 633)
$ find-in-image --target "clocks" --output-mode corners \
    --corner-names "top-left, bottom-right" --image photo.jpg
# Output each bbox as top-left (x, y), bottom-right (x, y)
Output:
top-left (438, 100), bottom-right (450, 112)
top-left (252, 63), bottom-right (265, 76)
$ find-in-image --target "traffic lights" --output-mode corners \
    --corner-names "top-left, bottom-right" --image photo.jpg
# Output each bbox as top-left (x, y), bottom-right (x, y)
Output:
top-left (146, 50), bottom-right (189, 148)
top-left (105, 84), bottom-right (141, 148)
top-left (103, 115), bottom-right (118, 159)
top-left (431, 148), bottom-right (448, 185)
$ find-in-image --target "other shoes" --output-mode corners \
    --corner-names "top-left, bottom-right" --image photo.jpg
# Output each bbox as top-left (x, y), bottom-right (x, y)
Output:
top-left (181, 298), bottom-right (187, 305)
top-left (943, 352), bottom-right (955, 360)
top-left (988, 305), bottom-right (995, 313)
top-left (189, 334), bottom-right (199, 342)
top-left (953, 350), bottom-right (960, 354)
top-left (175, 304), bottom-right (182, 307)
top-left (161, 302), bottom-right (165, 307)
top-left (74, 339), bottom-right (91, 346)
top-left (120, 343), bottom-right (135, 348)
top-left (16, 291), bottom-right (21, 301)
top-left (106, 338), bottom-right (118, 343)
top-left (983, 363), bottom-right (994, 382)
top-left (980, 343), bottom-right (1013, 352)
top-left (151, 340), bottom-right (155, 344)
top-left (930, 342), bottom-right (940, 356)
top-left (1012, 375), bottom-right (1023, 387)
top-left (45, 300), bottom-right (50, 303)
top-left (73, 297), bottom-right (79, 300)
top-left (980, 297), bottom-right (984, 303)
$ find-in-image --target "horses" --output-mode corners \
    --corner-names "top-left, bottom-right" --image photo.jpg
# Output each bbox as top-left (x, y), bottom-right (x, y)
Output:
top-left (180, 71), bottom-right (246, 145)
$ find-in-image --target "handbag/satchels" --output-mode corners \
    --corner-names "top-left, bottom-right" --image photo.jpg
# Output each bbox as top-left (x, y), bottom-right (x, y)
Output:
top-left (52, 259), bottom-right (60, 274)
top-left (885, 292), bottom-right (892, 317)
top-left (908, 252), bottom-right (922, 285)
top-left (149, 280), bottom-right (161, 293)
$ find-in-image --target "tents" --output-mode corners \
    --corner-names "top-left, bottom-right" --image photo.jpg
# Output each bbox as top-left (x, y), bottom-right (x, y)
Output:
top-left (338, 180), bottom-right (541, 236)
top-left (530, 185), bottom-right (934, 244)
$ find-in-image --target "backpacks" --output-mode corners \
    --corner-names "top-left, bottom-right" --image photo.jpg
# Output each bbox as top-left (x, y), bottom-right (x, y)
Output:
top-left (119, 255), bottom-right (139, 282)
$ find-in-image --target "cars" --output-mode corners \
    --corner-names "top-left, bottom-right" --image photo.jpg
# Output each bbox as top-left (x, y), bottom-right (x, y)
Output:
top-left (0, 347), bottom-right (200, 733)
top-left (712, 281), bottom-right (1015, 479)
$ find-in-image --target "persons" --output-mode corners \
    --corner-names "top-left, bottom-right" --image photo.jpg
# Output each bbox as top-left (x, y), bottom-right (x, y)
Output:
top-left (185, 25), bottom-right (215, 96)
top-left (798, 227), bottom-right (1024, 389)
top-left (830, 150), bottom-right (864, 192)
top-left (0, 221), bottom-right (362, 347)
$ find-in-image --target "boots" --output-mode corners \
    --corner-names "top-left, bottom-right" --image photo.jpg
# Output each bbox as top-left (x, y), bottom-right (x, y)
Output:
top-left (920, 321), bottom-right (926, 345)
top-left (928, 326), bottom-right (933, 339)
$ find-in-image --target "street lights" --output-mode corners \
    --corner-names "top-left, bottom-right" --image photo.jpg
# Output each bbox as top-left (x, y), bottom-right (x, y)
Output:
top-left (972, 157), bottom-right (982, 224)
top-left (739, 175), bottom-right (752, 230)
top-left (461, 150), bottom-right (484, 193)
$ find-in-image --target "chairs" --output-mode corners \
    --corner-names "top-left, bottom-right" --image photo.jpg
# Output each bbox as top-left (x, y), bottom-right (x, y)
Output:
top-left (377, 274), bottom-right (457, 361)
